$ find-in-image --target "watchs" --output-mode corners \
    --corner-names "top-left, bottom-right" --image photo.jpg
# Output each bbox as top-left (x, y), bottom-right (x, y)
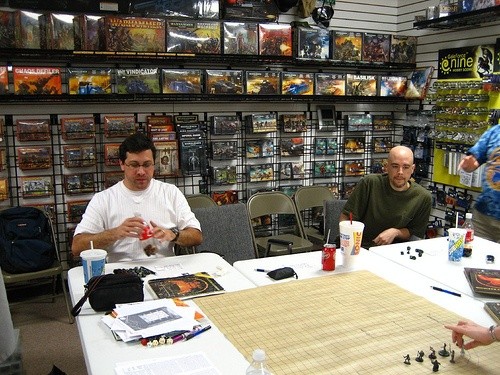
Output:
top-left (169, 226), bottom-right (179, 241)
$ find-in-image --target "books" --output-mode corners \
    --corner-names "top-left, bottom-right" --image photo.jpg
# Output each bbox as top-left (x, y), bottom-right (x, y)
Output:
top-left (147, 270), bottom-right (229, 301)
top-left (102, 299), bottom-right (202, 346)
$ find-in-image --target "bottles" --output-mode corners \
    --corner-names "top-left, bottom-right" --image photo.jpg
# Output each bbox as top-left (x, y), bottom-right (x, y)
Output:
top-left (245, 349), bottom-right (271, 375)
top-left (461, 213), bottom-right (474, 257)
top-left (134, 213), bottom-right (161, 256)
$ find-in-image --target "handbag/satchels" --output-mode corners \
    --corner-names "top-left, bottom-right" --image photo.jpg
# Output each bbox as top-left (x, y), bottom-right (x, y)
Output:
top-left (70, 272), bottom-right (143, 316)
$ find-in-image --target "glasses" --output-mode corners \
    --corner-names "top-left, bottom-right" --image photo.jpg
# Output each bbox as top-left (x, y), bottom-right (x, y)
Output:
top-left (123, 161), bottom-right (153, 168)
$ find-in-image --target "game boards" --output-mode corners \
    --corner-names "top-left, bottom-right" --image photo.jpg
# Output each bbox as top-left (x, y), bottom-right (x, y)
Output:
top-left (190, 269), bottom-right (499, 375)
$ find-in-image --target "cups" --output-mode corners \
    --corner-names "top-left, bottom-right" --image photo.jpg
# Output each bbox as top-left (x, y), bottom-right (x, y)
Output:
top-left (338, 221), bottom-right (364, 268)
top-left (448, 228), bottom-right (467, 263)
top-left (80, 249), bottom-right (107, 286)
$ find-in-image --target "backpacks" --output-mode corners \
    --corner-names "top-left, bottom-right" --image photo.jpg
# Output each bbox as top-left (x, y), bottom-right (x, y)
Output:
top-left (0, 207), bottom-right (56, 274)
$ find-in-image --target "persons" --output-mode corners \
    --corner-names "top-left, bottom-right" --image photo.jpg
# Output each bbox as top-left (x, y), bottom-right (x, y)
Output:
top-left (337, 146), bottom-right (431, 248)
top-left (456, 123), bottom-right (500, 243)
top-left (70, 134), bottom-right (203, 261)
top-left (443, 318), bottom-right (500, 351)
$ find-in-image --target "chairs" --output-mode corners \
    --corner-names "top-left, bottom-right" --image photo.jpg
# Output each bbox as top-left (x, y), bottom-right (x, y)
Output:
top-left (246, 192), bottom-right (313, 257)
top-left (324, 200), bottom-right (350, 243)
top-left (295, 185), bottom-right (331, 241)
top-left (190, 203), bottom-right (256, 266)
top-left (0, 205), bottom-right (75, 325)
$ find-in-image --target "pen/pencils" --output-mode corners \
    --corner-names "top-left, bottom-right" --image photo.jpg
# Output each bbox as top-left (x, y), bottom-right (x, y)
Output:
top-left (255, 267), bottom-right (270, 273)
top-left (182, 325), bottom-right (213, 341)
top-left (430, 285), bottom-right (460, 298)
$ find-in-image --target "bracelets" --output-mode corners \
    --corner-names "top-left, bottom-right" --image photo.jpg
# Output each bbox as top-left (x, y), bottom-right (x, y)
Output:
top-left (487, 325), bottom-right (497, 343)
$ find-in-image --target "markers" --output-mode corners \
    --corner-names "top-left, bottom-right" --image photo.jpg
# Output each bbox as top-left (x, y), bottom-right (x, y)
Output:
top-left (137, 329), bottom-right (190, 346)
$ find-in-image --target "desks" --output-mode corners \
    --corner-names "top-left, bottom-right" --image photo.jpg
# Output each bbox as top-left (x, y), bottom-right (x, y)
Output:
top-left (369, 235), bottom-right (500, 302)
top-left (66, 251), bottom-right (257, 375)
top-left (234, 249), bottom-right (500, 375)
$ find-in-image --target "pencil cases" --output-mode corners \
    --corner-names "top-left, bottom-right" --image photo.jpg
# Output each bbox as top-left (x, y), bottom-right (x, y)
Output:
top-left (267, 266), bottom-right (295, 279)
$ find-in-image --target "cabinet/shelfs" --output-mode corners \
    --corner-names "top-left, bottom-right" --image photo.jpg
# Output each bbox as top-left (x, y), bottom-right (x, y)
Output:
top-left (0, 0), bottom-right (434, 104)
top-left (0, 111), bottom-right (433, 262)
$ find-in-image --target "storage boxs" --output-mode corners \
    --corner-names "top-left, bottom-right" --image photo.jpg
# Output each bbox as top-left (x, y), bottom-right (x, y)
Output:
top-left (0, 0), bottom-right (435, 97)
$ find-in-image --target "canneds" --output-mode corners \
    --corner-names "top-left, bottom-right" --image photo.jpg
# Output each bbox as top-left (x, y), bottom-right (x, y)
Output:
top-left (322, 243), bottom-right (336, 270)
